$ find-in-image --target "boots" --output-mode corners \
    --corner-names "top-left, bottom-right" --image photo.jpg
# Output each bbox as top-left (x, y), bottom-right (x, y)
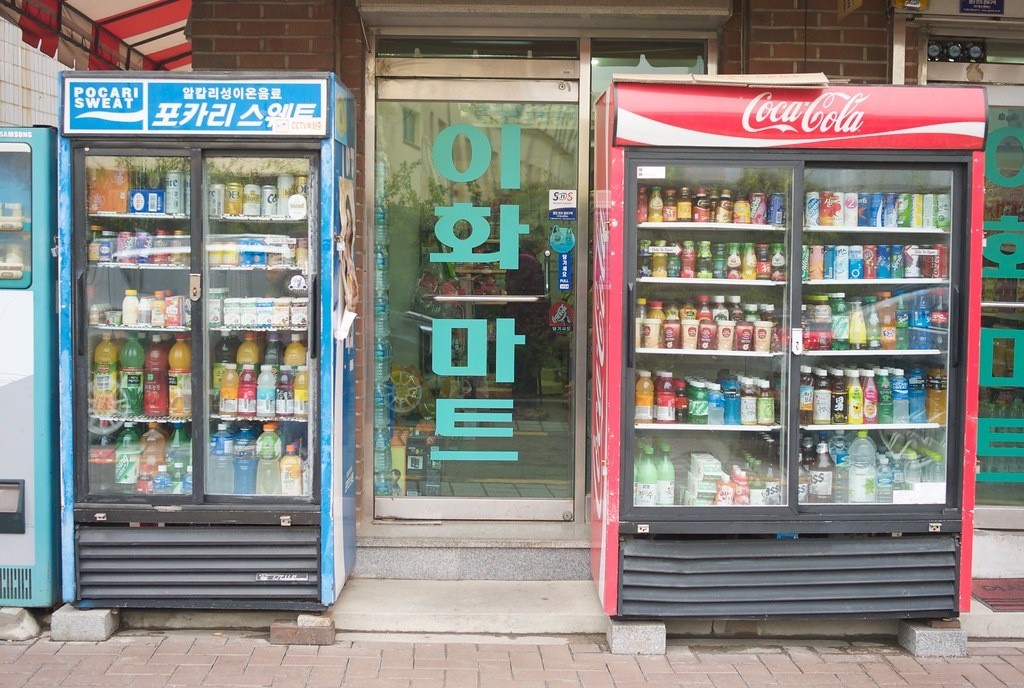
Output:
top-left (529, 391), bottom-right (550, 418)
top-left (512, 391), bottom-right (545, 420)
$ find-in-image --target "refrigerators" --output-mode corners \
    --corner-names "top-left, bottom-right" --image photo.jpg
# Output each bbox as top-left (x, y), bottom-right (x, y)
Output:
top-left (596, 77), bottom-right (989, 629)
top-left (59, 69), bottom-right (356, 612)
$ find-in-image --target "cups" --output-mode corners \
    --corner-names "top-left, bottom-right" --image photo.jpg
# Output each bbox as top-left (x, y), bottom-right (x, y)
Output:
top-left (754, 321), bottom-right (773, 353)
top-left (635, 318), bottom-right (642, 348)
top-left (717, 321), bottom-right (735, 351)
top-left (680, 320), bottom-right (699, 349)
top-left (736, 321), bottom-right (753, 351)
top-left (643, 319), bottom-right (661, 348)
top-left (663, 319), bottom-right (680, 348)
top-left (698, 321), bottom-right (717, 350)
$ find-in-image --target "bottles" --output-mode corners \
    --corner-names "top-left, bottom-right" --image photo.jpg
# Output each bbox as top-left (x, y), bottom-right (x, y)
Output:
top-left (375, 384), bottom-right (390, 428)
top-left (86, 225), bottom-right (190, 265)
top-left (375, 290), bottom-right (390, 338)
top-left (978, 399), bottom-right (1024, 482)
top-left (375, 429), bottom-right (392, 496)
top-left (636, 295), bottom-right (782, 352)
top-left (374, 200), bottom-right (391, 245)
top-left (209, 420), bottom-right (307, 497)
top-left (375, 338), bottom-right (393, 383)
top-left (637, 239), bottom-right (785, 281)
top-left (376, 151), bottom-right (390, 199)
top-left (801, 289), bottom-right (949, 349)
top-left (638, 185), bottom-right (732, 223)
top-left (89, 289), bottom-right (172, 326)
top-left (374, 245), bottom-right (390, 290)
top-left (95, 331), bottom-right (191, 416)
top-left (634, 367), bottom-right (781, 506)
top-left (88, 420), bottom-right (193, 495)
top-left (211, 331), bottom-right (309, 419)
top-left (797, 363), bottom-right (946, 504)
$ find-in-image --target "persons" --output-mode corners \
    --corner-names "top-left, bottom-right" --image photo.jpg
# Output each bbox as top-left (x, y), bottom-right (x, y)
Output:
top-left (504, 221), bottom-right (552, 421)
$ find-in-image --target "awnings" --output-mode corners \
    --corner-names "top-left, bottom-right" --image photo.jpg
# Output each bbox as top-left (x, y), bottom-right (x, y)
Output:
top-left (0, 0), bottom-right (193, 72)
